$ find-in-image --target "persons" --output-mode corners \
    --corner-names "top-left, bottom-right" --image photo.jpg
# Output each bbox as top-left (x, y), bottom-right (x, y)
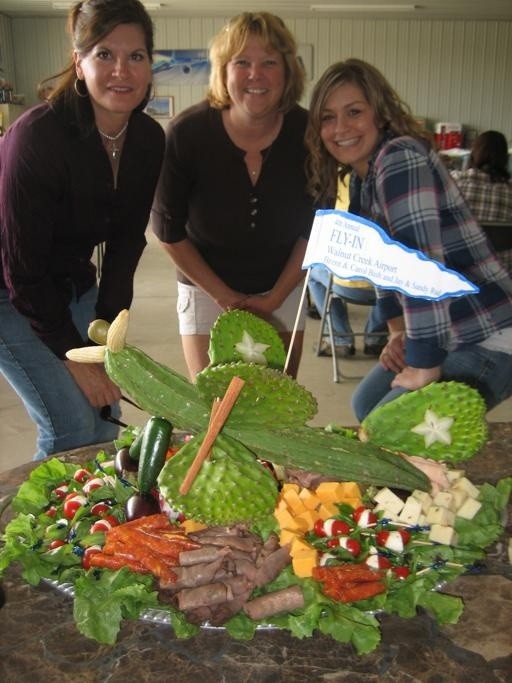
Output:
top-left (309, 161), bottom-right (390, 356)
top-left (0, 0), bottom-right (165, 463)
top-left (151, 9), bottom-right (338, 384)
top-left (303, 55), bottom-right (511, 424)
top-left (451, 129), bottom-right (512, 273)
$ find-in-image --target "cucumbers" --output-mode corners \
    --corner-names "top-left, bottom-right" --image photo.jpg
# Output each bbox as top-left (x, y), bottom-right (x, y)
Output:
top-left (105, 338), bottom-right (431, 493)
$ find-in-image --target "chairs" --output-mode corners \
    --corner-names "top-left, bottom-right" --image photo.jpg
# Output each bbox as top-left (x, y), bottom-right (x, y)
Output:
top-left (436, 123), bottom-right (471, 171)
top-left (315, 274), bottom-right (389, 383)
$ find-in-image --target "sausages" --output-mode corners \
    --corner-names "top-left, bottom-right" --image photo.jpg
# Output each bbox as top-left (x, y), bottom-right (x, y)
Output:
top-left (159, 522), bottom-right (304, 622)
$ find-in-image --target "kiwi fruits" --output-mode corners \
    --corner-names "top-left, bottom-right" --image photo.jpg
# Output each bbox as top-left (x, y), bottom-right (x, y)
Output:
top-left (87, 319), bottom-right (110, 346)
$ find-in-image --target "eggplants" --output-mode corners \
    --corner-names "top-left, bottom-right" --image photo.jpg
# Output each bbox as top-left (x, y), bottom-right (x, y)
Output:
top-left (125, 494), bottom-right (158, 522)
top-left (114, 446), bottom-right (139, 486)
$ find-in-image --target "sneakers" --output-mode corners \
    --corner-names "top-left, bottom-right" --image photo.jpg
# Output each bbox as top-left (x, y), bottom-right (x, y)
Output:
top-left (363, 344), bottom-right (385, 356)
top-left (314, 341), bottom-right (355, 356)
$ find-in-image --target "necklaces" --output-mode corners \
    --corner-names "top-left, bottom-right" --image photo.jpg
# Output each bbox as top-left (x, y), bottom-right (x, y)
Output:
top-left (228, 115), bottom-right (261, 176)
top-left (98, 119), bottom-right (129, 159)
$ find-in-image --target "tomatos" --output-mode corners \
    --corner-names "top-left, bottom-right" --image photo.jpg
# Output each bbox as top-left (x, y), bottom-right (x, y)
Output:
top-left (46, 468), bottom-right (118, 570)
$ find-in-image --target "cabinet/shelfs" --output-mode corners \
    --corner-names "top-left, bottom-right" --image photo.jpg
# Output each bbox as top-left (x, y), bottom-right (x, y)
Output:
top-left (0, 104), bottom-right (29, 140)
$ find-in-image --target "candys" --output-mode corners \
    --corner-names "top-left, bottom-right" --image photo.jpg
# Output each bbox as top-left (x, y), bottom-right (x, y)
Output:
top-left (313, 508), bottom-right (411, 582)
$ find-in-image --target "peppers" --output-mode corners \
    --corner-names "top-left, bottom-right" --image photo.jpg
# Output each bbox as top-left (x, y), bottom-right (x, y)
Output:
top-left (129, 416), bottom-right (172, 498)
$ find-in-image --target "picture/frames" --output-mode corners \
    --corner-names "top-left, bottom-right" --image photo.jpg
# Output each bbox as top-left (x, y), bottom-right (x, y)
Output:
top-left (143, 96), bottom-right (174, 119)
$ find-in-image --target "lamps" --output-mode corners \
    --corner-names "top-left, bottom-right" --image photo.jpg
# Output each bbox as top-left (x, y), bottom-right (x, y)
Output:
top-left (310, 5), bottom-right (415, 13)
top-left (52, 2), bottom-right (161, 10)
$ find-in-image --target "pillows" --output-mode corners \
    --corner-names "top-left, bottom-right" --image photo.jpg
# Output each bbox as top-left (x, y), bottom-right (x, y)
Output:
top-left (434, 131), bottom-right (462, 151)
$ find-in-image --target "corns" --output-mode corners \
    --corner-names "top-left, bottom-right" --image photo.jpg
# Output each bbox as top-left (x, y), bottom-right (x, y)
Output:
top-left (65, 346), bottom-right (105, 364)
top-left (106, 307), bottom-right (130, 354)
